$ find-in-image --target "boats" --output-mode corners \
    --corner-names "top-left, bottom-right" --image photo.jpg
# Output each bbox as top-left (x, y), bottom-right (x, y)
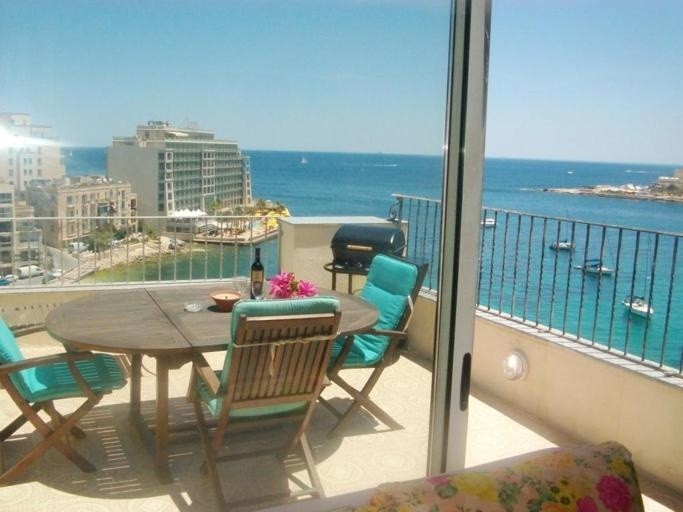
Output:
top-left (479, 217), bottom-right (500, 228)
top-left (621, 294), bottom-right (655, 320)
top-left (572, 257), bottom-right (614, 277)
top-left (548, 239), bottom-right (575, 251)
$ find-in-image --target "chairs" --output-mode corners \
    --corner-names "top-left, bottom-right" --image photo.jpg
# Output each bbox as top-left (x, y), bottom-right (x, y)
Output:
top-left (318, 252), bottom-right (429, 442)
top-left (0, 316), bottom-right (127, 486)
top-left (185, 297), bottom-right (342, 512)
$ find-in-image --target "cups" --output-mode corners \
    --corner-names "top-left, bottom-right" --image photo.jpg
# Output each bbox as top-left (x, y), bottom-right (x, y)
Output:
top-left (233, 277), bottom-right (248, 295)
top-left (251, 282), bottom-right (266, 300)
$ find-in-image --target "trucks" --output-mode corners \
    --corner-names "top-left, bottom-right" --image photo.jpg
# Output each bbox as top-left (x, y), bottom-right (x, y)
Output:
top-left (66, 242), bottom-right (84, 254)
top-left (16, 265), bottom-right (45, 279)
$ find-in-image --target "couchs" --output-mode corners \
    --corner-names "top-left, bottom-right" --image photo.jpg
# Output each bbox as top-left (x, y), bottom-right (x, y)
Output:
top-left (191, 438), bottom-right (653, 512)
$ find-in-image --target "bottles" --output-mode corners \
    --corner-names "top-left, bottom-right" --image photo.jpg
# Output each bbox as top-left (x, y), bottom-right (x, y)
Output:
top-left (249, 245), bottom-right (263, 300)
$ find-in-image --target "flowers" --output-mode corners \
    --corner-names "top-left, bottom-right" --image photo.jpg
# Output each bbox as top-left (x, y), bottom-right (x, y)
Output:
top-left (265, 272), bottom-right (317, 299)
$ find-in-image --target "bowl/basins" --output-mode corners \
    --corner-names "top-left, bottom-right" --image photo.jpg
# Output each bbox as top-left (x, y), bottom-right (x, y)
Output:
top-left (209, 291), bottom-right (240, 312)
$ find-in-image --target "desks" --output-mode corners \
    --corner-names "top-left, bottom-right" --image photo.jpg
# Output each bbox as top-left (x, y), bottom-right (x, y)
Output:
top-left (46, 278), bottom-right (379, 488)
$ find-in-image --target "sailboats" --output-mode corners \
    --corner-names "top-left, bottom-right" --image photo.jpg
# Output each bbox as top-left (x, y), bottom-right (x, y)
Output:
top-left (300, 156), bottom-right (308, 164)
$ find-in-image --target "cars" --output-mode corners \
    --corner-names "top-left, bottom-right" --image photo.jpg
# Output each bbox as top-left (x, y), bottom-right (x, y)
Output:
top-left (0, 280), bottom-right (10, 286)
top-left (5, 274), bottom-right (20, 282)
top-left (51, 268), bottom-right (65, 279)
